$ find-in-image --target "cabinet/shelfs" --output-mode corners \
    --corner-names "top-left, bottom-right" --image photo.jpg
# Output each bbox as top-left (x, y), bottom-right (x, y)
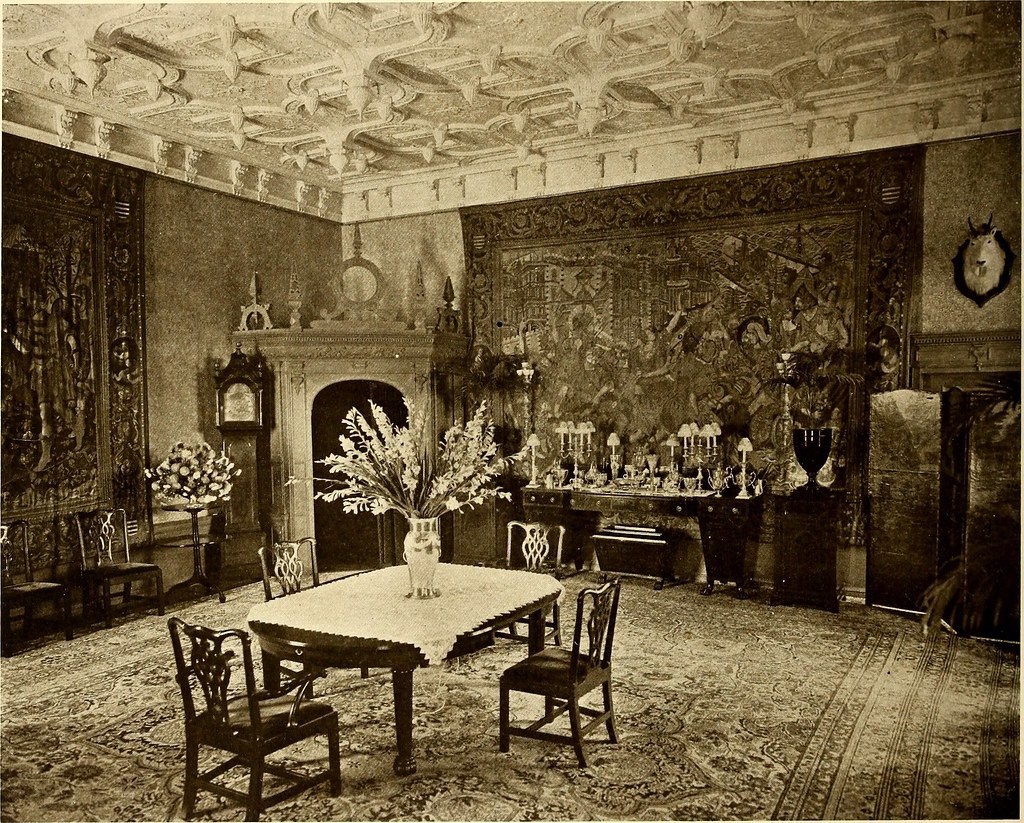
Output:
top-left (773, 493), bottom-right (843, 612)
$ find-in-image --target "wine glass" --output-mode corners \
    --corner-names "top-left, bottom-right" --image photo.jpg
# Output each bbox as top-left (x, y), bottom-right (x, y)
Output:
top-left (652, 477), bottom-right (661, 495)
top-left (552, 470), bottom-right (567, 489)
top-left (597, 473), bottom-right (607, 491)
top-left (683, 478), bottom-right (696, 495)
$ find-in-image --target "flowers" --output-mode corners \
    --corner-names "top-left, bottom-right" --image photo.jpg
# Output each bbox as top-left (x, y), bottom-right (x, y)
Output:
top-left (144, 438), bottom-right (243, 502)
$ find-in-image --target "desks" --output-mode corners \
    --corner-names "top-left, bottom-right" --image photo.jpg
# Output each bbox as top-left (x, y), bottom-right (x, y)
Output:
top-left (245, 559), bottom-right (567, 778)
top-left (520, 479), bottom-right (765, 599)
top-left (159, 500), bottom-right (232, 603)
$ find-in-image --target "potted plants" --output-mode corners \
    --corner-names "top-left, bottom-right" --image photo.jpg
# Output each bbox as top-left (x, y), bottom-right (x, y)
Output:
top-left (757, 346), bottom-right (867, 493)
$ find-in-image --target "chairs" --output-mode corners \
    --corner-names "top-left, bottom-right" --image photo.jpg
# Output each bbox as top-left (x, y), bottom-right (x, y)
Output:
top-left (499, 573), bottom-right (623, 767)
top-left (1, 519), bottom-right (74, 658)
top-left (72, 507), bottom-right (165, 629)
top-left (488, 520), bottom-right (566, 646)
top-left (166, 616), bottom-right (342, 822)
top-left (257, 536), bottom-right (369, 700)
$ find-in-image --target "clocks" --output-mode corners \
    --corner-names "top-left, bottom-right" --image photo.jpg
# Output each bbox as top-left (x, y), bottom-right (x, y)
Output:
top-left (213, 340), bottom-right (265, 431)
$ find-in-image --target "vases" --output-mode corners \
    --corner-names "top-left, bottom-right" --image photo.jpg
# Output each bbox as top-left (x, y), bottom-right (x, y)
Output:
top-left (287, 392), bottom-right (527, 600)
top-left (180, 492), bottom-right (204, 509)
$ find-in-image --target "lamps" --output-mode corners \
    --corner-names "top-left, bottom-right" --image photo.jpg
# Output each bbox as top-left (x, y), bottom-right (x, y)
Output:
top-left (526, 422), bottom-right (757, 499)
